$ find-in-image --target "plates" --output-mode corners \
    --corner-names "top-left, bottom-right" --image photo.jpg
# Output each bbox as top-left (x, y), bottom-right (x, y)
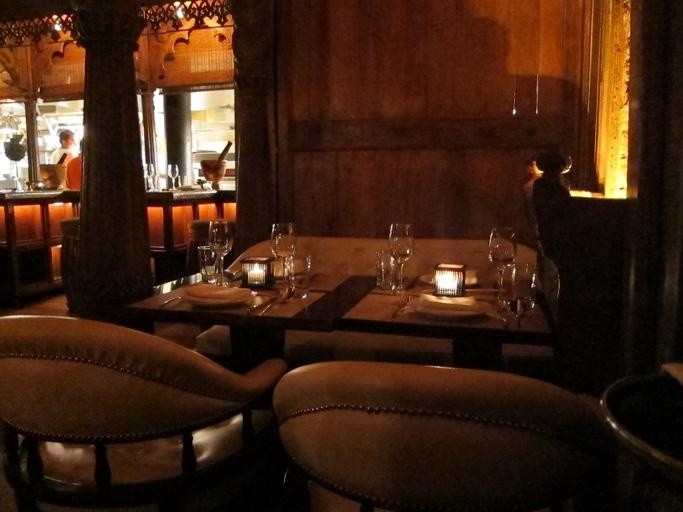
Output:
top-left (403, 299), bottom-right (491, 318)
top-left (418, 273), bottom-right (478, 287)
top-left (175, 283), bottom-right (252, 309)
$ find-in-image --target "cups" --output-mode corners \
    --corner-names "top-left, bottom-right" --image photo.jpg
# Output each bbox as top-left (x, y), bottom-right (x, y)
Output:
top-left (288, 253), bottom-right (311, 291)
top-left (511, 261), bottom-right (538, 310)
top-left (375, 248), bottom-right (395, 289)
top-left (197, 246), bottom-right (219, 283)
top-left (207, 180), bottom-right (212, 190)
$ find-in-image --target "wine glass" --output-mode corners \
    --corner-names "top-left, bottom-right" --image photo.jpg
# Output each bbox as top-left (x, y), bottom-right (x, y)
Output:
top-left (143, 163), bottom-right (155, 192)
top-left (197, 159), bottom-right (228, 191)
top-left (11, 176), bottom-right (30, 192)
top-left (487, 226), bottom-right (517, 308)
top-left (167, 163), bottom-right (178, 191)
top-left (208, 218), bottom-right (235, 284)
top-left (387, 222), bottom-right (416, 295)
top-left (270, 222), bottom-right (308, 302)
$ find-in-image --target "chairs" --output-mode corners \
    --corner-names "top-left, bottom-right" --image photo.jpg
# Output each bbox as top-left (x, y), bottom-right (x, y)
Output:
top-left (0, 311), bottom-right (288, 512)
top-left (270, 361), bottom-right (615, 512)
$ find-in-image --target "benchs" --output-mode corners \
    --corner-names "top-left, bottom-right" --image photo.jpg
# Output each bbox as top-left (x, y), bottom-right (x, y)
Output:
top-left (192, 233), bottom-right (557, 376)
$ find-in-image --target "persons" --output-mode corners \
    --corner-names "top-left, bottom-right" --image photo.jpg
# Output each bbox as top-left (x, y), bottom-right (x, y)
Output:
top-left (67, 139), bottom-right (83, 190)
top-left (50, 130), bottom-right (76, 190)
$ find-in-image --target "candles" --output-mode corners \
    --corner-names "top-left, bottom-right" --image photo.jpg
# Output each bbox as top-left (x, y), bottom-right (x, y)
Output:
top-left (247, 268), bottom-right (265, 284)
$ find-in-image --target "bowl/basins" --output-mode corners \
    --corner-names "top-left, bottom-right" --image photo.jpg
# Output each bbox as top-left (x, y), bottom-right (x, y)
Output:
top-left (26, 180), bottom-right (44, 191)
top-left (38, 163), bottom-right (65, 189)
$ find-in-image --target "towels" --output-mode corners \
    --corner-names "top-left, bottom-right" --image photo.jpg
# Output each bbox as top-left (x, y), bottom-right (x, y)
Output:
top-left (399, 290), bottom-right (486, 319)
top-left (183, 283), bottom-right (251, 304)
